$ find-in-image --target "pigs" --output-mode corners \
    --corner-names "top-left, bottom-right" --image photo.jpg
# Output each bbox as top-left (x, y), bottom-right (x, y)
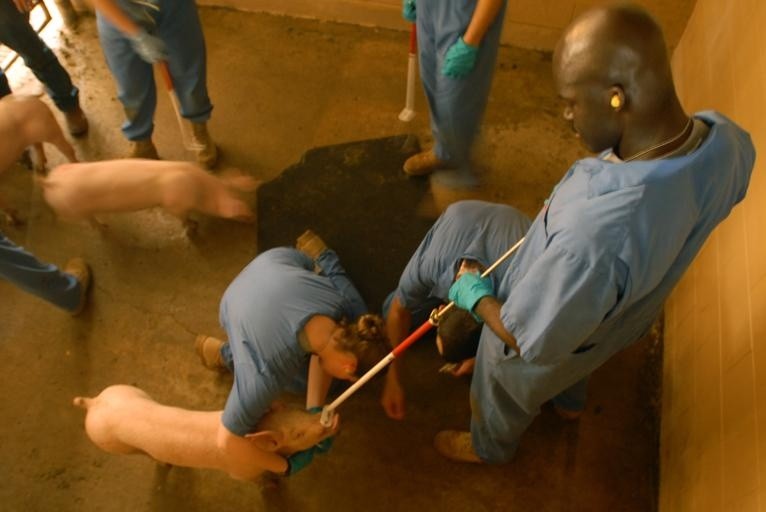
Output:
top-left (0, 90), bottom-right (81, 174)
top-left (72, 383), bottom-right (342, 490)
top-left (31, 158), bottom-right (266, 226)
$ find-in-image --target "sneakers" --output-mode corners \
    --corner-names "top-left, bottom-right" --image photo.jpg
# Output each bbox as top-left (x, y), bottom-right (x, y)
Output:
top-left (64, 107), bottom-right (88, 138)
top-left (294, 230), bottom-right (328, 274)
top-left (191, 335), bottom-right (227, 377)
top-left (62, 256), bottom-right (92, 318)
top-left (404, 149), bottom-right (459, 178)
top-left (554, 399), bottom-right (583, 420)
top-left (434, 430), bottom-right (503, 463)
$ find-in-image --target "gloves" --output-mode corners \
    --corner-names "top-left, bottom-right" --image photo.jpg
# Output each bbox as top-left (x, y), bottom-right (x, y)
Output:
top-left (131, 32), bottom-right (173, 64)
top-left (305, 403), bottom-right (336, 456)
top-left (285, 442), bottom-right (315, 477)
top-left (402, 0), bottom-right (417, 21)
top-left (441, 37), bottom-right (480, 82)
top-left (449, 270), bottom-right (498, 323)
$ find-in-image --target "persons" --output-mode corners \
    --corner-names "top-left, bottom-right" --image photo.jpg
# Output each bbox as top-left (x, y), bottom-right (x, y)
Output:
top-left (0, 0), bottom-right (89, 171)
top-left (431, 4), bottom-right (756, 465)
top-left (0, 236), bottom-right (94, 318)
top-left (381, 199), bottom-right (531, 376)
top-left (193, 228), bottom-right (393, 476)
top-left (402, 0), bottom-right (505, 177)
top-left (83, 0), bottom-right (219, 171)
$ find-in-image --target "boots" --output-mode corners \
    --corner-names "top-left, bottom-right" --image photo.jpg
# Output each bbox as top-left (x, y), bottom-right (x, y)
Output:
top-left (121, 140), bottom-right (161, 159)
top-left (185, 121), bottom-right (219, 169)
